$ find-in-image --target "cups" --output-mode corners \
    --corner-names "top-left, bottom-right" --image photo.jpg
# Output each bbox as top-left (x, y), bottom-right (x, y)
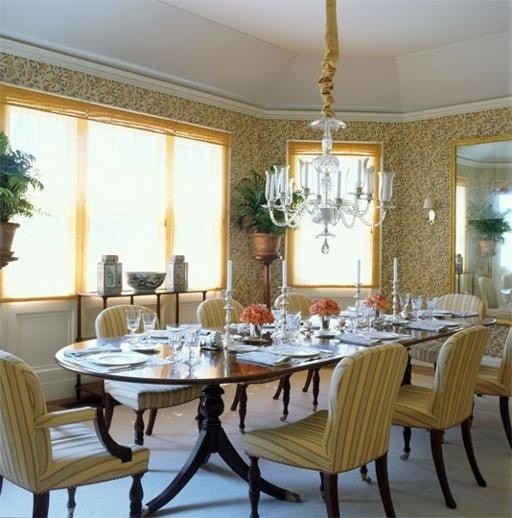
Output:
top-left (478, 300), bottom-right (487, 321)
top-left (346, 306), bottom-right (359, 328)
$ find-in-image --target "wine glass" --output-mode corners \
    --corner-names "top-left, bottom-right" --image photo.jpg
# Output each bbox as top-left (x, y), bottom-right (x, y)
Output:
top-left (272, 307), bottom-right (302, 339)
top-left (166, 322), bottom-right (201, 365)
top-left (125, 308), bottom-right (156, 344)
top-left (397, 292), bottom-right (438, 318)
top-left (168, 366), bottom-right (201, 380)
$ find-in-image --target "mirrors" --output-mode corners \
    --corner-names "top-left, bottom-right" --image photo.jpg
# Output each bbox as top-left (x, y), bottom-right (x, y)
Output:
top-left (449, 132), bottom-right (511, 328)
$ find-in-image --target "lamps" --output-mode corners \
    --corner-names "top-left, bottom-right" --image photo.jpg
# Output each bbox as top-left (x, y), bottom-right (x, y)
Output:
top-left (257, 1), bottom-right (400, 255)
top-left (420, 195), bottom-right (439, 226)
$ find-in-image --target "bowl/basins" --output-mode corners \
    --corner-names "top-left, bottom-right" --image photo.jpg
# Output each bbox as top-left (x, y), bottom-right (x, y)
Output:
top-left (126, 271), bottom-right (167, 293)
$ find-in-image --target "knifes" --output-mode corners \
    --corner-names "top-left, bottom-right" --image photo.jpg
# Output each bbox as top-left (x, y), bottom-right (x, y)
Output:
top-left (304, 353), bottom-right (335, 363)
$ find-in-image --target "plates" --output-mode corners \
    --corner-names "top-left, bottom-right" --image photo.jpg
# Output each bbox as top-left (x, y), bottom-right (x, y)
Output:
top-left (89, 351), bottom-right (149, 366)
top-left (432, 320), bottom-right (461, 327)
top-left (434, 310), bottom-right (450, 318)
top-left (362, 329), bottom-right (398, 340)
top-left (226, 344), bottom-right (258, 353)
top-left (271, 346), bottom-right (319, 356)
top-left (149, 327), bottom-right (170, 339)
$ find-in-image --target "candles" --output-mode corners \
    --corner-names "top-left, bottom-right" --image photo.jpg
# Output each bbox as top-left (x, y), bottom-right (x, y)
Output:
top-left (357, 260), bottom-right (361, 282)
top-left (226, 260), bottom-right (233, 290)
top-left (282, 260), bottom-right (288, 287)
top-left (394, 256), bottom-right (397, 281)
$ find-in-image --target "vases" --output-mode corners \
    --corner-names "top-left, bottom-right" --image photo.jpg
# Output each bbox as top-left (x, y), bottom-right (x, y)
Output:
top-left (454, 249), bottom-right (464, 277)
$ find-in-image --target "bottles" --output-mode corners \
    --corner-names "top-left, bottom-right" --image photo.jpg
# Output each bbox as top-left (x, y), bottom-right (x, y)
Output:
top-left (97, 255), bottom-right (122, 296)
top-left (168, 254), bottom-right (189, 292)
top-left (383, 318), bottom-right (392, 331)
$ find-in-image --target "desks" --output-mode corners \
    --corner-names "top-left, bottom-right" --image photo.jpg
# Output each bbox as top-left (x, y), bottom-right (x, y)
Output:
top-left (51, 306), bottom-right (479, 468)
top-left (454, 271), bottom-right (478, 297)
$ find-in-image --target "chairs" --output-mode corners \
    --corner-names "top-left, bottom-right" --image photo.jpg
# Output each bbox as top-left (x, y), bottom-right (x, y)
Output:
top-left (240, 341), bottom-right (411, 516)
top-left (271, 290), bottom-right (325, 412)
top-left (433, 326), bottom-right (511, 452)
top-left (92, 302), bottom-right (214, 472)
top-left (1, 347), bottom-right (148, 516)
top-left (429, 293), bottom-right (487, 326)
top-left (194, 295), bottom-right (293, 435)
top-left (359, 325), bottom-right (494, 509)
top-left (477, 275), bottom-right (511, 322)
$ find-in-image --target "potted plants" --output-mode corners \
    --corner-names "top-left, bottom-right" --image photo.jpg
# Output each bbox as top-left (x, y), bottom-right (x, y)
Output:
top-left (463, 190), bottom-right (511, 253)
top-left (226, 159), bottom-right (305, 255)
top-left (364, 295), bottom-right (390, 332)
top-left (306, 296), bottom-right (343, 333)
top-left (238, 301), bottom-right (276, 339)
top-left (0, 128), bottom-right (46, 270)
